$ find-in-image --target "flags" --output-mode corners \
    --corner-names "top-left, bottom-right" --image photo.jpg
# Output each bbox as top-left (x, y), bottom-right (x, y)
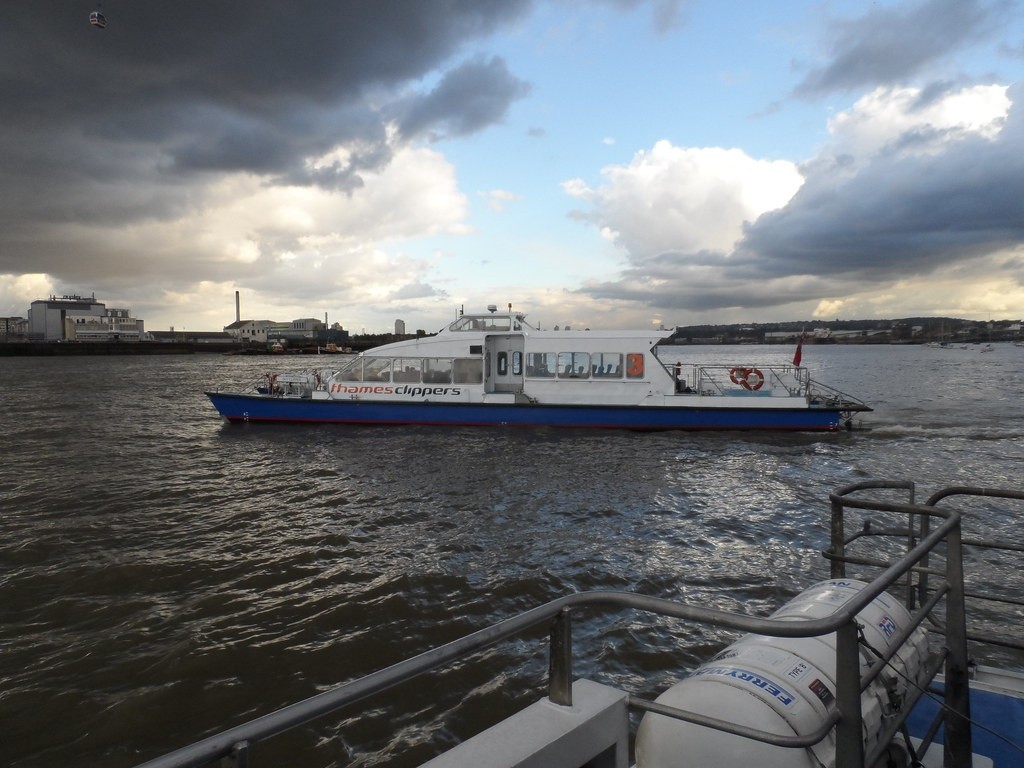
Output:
top-left (792, 338), bottom-right (803, 367)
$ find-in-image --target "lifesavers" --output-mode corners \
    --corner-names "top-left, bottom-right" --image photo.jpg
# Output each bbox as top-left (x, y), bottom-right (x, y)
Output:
top-left (741, 369), bottom-right (764, 390)
top-left (730, 368), bottom-right (748, 384)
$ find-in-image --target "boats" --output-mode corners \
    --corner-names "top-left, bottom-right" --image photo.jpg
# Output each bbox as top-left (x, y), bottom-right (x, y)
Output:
top-left (198, 300), bottom-right (879, 435)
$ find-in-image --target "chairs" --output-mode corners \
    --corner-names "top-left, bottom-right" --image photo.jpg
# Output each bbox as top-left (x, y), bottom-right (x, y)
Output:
top-left (382, 366), bottom-right (451, 383)
top-left (560, 364), bottom-right (622, 378)
